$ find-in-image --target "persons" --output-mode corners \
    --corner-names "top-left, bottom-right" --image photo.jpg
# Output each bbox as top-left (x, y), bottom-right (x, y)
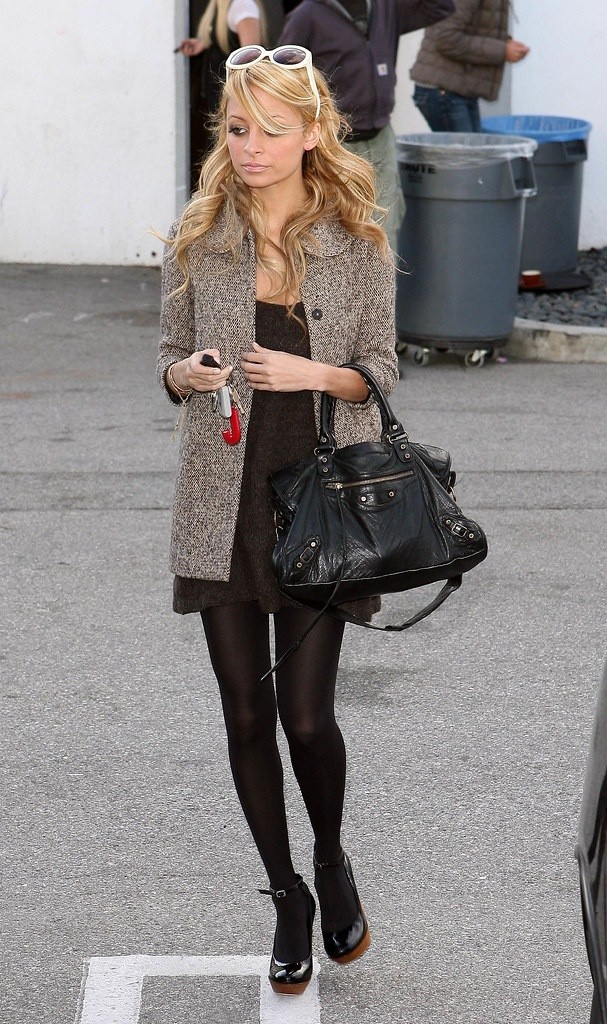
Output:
top-left (278, 1), bottom-right (401, 261)
top-left (411, 0), bottom-right (529, 133)
top-left (181, 0), bottom-right (268, 145)
top-left (155, 46), bottom-right (400, 994)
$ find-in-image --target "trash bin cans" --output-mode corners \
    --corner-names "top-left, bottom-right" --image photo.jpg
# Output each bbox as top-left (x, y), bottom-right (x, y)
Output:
top-left (480, 113), bottom-right (592, 285)
top-left (395, 132), bottom-right (539, 369)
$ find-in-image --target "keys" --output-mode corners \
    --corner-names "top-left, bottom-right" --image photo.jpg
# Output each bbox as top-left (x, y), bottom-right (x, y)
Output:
top-left (200, 354), bottom-right (222, 369)
top-left (207, 379), bottom-right (246, 445)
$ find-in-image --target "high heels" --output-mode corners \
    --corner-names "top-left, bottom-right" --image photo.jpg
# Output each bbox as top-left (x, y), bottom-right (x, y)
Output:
top-left (257, 874), bottom-right (316, 996)
top-left (314, 840), bottom-right (371, 964)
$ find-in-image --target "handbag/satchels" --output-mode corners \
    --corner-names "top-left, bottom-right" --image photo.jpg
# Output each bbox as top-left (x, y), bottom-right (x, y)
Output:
top-left (261, 362), bottom-right (488, 682)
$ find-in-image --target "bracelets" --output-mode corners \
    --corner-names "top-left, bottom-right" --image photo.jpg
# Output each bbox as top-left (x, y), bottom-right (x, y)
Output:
top-left (166, 362), bottom-right (194, 438)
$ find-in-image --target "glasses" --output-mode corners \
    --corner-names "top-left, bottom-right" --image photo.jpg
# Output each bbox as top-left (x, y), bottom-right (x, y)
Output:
top-left (225, 45), bottom-right (321, 120)
